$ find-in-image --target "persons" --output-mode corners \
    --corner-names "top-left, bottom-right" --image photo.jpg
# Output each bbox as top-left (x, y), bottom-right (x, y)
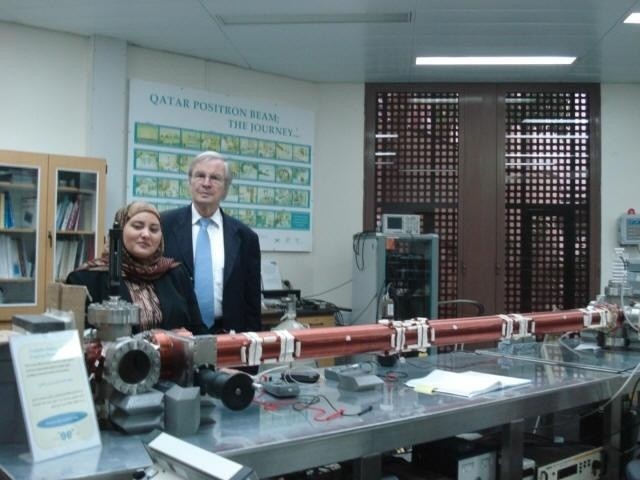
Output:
top-left (158, 150), bottom-right (264, 334)
top-left (67, 198), bottom-right (209, 336)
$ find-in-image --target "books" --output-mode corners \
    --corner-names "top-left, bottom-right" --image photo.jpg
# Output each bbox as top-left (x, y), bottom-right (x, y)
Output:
top-left (0, 182), bottom-right (89, 286)
top-left (403, 365), bottom-right (533, 400)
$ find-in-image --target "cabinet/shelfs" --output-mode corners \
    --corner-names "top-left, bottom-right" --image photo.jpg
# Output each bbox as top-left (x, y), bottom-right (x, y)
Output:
top-left (0, 149), bottom-right (640, 480)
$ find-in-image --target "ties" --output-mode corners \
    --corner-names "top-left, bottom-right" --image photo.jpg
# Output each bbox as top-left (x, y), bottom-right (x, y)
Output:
top-left (193, 219), bottom-right (216, 330)
